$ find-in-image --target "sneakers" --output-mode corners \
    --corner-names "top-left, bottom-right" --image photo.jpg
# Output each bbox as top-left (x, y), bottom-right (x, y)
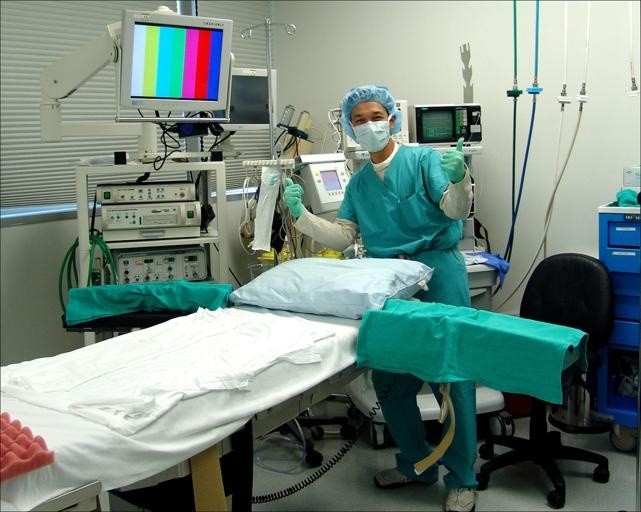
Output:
top-left (445, 487), bottom-right (475, 512)
top-left (374, 469), bottom-right (438, 490)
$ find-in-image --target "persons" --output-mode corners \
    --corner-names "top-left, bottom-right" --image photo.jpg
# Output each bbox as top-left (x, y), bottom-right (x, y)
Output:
top-left (283, 86), bottom-right (481, 512)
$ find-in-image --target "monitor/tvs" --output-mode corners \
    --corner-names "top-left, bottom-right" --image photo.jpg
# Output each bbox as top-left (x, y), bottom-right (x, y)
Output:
top-left (215, 67), bottom-right (278, 131)
top-left (120, 9), bottom-right (233, 111)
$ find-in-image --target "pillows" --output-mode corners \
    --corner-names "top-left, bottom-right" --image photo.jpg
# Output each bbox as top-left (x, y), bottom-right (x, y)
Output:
top-left (233, 253), bottom-right (434, 320)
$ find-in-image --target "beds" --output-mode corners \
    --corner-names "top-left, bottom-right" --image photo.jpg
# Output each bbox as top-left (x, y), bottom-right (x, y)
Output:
top-left (0, 306), bottom-right (372, 512)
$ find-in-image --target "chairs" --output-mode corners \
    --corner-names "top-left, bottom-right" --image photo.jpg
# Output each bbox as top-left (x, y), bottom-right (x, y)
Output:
top-left (475, 253), bottom-right (611, 511)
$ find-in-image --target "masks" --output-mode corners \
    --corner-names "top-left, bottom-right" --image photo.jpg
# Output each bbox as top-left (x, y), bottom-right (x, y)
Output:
top-left (349, 113), bottom-right (392, 152)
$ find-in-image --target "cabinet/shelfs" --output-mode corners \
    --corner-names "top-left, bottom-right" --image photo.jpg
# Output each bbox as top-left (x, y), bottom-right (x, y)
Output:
top-left (76, 158), bottom-right (228, 343)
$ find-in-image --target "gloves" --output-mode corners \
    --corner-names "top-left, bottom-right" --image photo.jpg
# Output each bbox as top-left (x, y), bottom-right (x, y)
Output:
top-left (440, 137), bottom-right (465, 182)
top-left (282, 178), bottom-right (304, 220)
